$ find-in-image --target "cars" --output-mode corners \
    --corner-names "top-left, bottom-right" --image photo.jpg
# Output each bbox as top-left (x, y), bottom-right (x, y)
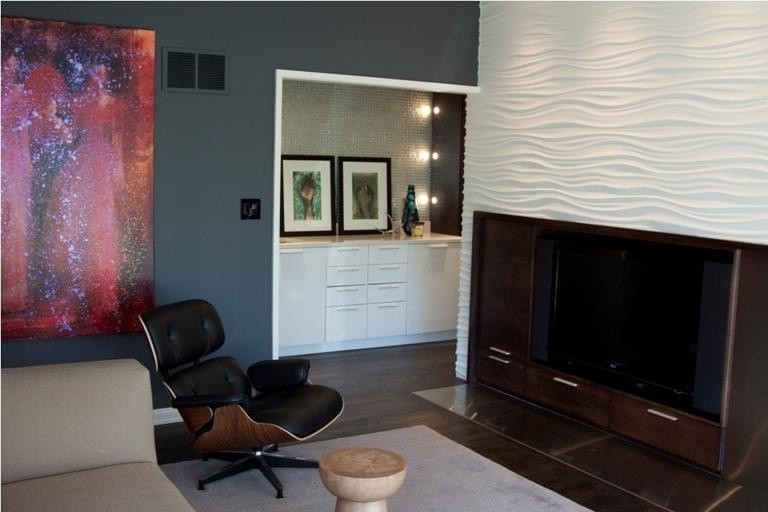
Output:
top-left (409, 221), bottom-right (425, 237)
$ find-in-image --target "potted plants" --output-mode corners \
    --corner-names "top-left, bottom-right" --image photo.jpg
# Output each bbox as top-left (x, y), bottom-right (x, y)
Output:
top-left (282, 154), bottom-right (335, 236)
top-left (338, 156), bottom-right (392, 234)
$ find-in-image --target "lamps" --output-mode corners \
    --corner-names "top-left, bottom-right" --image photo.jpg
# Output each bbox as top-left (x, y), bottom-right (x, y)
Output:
top-left (139, 299), bottom-right (344, 498)
top-left (1, 358), bottom-right (195, 511)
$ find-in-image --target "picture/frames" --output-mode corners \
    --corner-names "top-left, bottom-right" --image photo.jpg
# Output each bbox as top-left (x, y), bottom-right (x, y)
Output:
top-left (412, 385), bottom-right (750, 512)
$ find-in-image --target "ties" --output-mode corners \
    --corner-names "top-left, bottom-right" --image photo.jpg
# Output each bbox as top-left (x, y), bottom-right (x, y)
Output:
top-left (546, 232), bottom-right (704, 407)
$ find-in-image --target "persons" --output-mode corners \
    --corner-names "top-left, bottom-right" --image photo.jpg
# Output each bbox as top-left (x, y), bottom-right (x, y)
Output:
top-left (298, 172), bottom-right (315, 220)
top-left (357, 182), bottom-right (373, 219)
top-left (49, 61), bottom-right (123, 318)
top-left (1, 52), bottom-right (58, 312)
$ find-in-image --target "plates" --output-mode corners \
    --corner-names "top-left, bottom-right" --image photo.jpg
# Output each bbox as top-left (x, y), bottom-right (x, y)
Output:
top-left (523, 361), bottom-right (724, 473)
top-left (469, 211), bottom-right (532, 401)
top-left (279, 233), bottom-right (462, 357)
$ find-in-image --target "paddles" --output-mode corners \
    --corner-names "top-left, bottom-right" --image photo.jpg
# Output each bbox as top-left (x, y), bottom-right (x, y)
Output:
top-left (159, 424), bottom-right (595, 512)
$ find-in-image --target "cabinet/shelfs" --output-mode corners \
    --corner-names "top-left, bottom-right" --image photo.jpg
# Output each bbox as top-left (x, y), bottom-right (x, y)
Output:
top-left (400, 184), bottom-right (419, 236)
